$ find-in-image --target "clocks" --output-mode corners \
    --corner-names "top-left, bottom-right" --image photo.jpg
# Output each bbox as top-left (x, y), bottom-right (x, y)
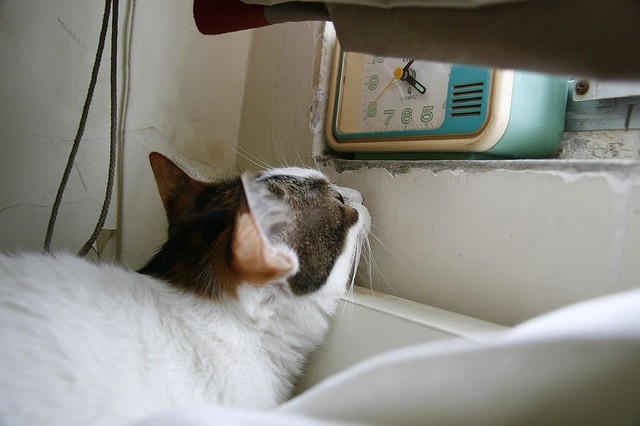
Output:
top-left (325, 34), bottom-right (568, 158)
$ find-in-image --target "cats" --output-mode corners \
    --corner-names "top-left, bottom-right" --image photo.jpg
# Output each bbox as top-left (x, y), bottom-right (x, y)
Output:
top-left (0, 151), bottom-right (375, 426)
